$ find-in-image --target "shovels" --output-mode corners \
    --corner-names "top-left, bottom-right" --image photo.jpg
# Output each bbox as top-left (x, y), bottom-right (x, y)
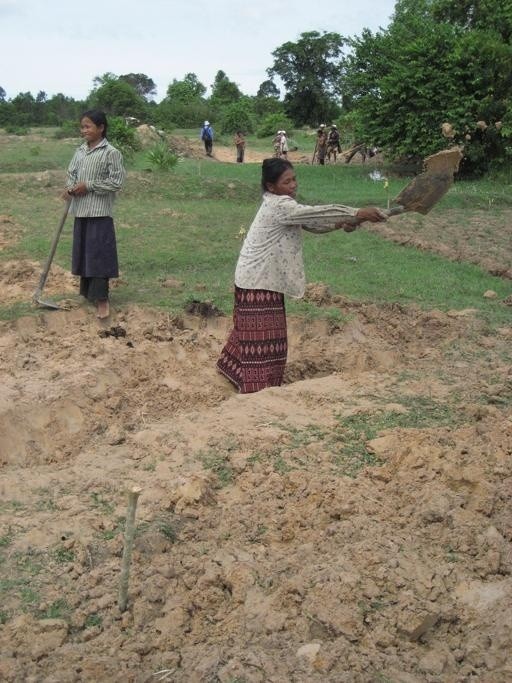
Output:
top-left (341, 172), bottom-right (454, 226)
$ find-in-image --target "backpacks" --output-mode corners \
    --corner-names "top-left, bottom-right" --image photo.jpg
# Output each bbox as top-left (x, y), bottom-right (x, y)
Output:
top-left (203, 129), bottom-right (210, 141)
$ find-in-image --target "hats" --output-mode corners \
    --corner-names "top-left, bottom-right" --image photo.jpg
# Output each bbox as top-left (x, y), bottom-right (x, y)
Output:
top-left (204, 121), bottom-right (209, 125)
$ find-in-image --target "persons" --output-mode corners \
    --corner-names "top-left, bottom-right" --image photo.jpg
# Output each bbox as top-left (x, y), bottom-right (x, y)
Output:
top-left (317, 122), bottom-right (328, 140)
top-left (313, 129), bottom-right (326, 165)
top-left (325, 124), bottom-right (340, 164)
top-left (200, 119), bottom-right (214, 157)
top-left (278, 130), bottom-right (288, 160)
top-left (63, 107), bottom-right (125, 321)
top-left (234, 131), bottom-right (245, 163)
top-left (271, 129), bottom-right (281, 158)
top-left (214, 154), bottom-right (389, 395)
